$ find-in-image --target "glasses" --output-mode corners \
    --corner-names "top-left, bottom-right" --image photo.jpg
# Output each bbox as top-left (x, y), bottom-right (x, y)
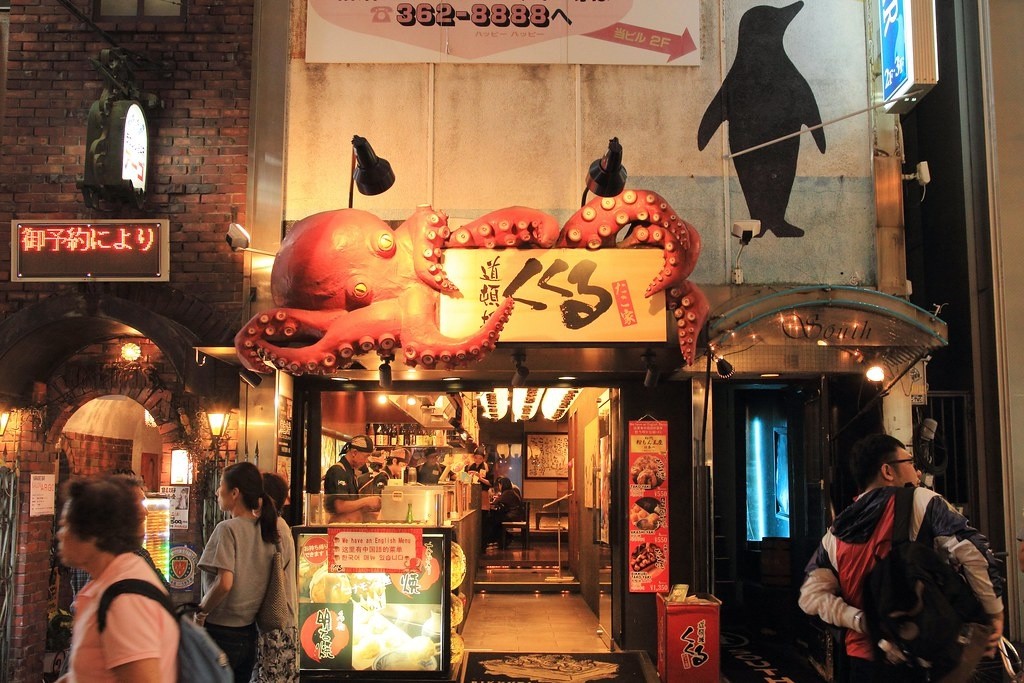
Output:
top-left (888, 459), bottom-right (915, 465)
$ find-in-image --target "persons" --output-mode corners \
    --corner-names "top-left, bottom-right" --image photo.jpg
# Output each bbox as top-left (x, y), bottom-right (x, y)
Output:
top-left (799, 432), bottom-right (1004, 683)
top-left (191, 461), bottom-right (277, 683)
top-left (55, 481), bottom-right (180, 683)
top-left (250, 472), bottom-right (301, 683)
top-left (68, 474), bottom-right (172, 608)
top-left (349, 446), bottom-right (522, 551)
top-left (324, 435), bottom-right (383, 523)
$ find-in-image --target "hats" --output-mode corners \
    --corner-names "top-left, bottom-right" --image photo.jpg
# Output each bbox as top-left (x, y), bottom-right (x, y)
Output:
top-left (474, 446), bottom-right (485, 457)
top-left (424, 447), bottom-right (436, 456)
top-left (349, 435), bottom-right (373, 452)
top-left (389, 448), bottom-right (410, 463)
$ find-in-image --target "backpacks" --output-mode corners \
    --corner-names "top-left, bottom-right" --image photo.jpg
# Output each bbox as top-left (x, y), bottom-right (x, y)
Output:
top-left (863, 482), bottom-right (970, 682)
top-left (98, 578), bottom-right (234, 683)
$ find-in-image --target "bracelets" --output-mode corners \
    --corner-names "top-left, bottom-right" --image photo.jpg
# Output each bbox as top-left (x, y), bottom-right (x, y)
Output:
top-left (852, 612), bottom-right (864, 633)
top-left (194, 603), bottom-right (208, 623)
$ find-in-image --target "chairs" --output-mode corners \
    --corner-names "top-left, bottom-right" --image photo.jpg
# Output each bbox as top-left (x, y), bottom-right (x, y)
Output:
top-left (500, 500), bottom-right (530, 552)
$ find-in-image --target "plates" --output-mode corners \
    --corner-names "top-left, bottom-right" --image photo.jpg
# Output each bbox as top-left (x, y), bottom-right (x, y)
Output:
top-left (351, 614), bottom-right (414, 670)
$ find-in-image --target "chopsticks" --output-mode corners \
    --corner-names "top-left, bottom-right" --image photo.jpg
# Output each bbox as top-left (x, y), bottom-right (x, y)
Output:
top-left (418, 642), bottom-right (441, 658)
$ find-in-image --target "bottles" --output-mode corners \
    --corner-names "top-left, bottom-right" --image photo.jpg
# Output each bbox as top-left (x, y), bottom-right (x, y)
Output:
top-left (405, 504), bottom-right (413, 523)
top-left (421, 610), bottom-right (441, 644)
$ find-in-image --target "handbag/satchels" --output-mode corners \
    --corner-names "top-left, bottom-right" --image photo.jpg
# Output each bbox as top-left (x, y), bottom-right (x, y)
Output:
top-left (255, 553), bottom-right (286, 636)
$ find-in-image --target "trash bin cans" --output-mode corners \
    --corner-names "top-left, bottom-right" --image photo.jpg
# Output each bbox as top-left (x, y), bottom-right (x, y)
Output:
top-left (656, 590), bottom-right (723, 683)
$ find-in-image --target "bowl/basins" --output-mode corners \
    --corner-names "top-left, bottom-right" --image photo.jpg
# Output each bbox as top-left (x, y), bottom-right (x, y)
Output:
top-left (372, 649), bottom-right (438, 671)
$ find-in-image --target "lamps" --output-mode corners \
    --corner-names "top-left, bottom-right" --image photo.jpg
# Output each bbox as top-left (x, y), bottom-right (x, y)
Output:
top-left (581, 137), bottom-right (627, 207)
top-left (349, 135), bottom-right (395, 208)
top-left (378, 354), bottom-right (394, 389)
top-left (226, 224), bottom-right (277, 258)
top-left (640, 353), bottom-right (661, 388)
top-left (511, 354), bottom-right (529, 389)
top-left (206, 391), bottom-right (232, 447)
top-left (238, 367), bottom-right (263, 389)
top-left (715, 356), bottom-right (735, 379)
top-left (732, 220), bottom-right (761, 284)
